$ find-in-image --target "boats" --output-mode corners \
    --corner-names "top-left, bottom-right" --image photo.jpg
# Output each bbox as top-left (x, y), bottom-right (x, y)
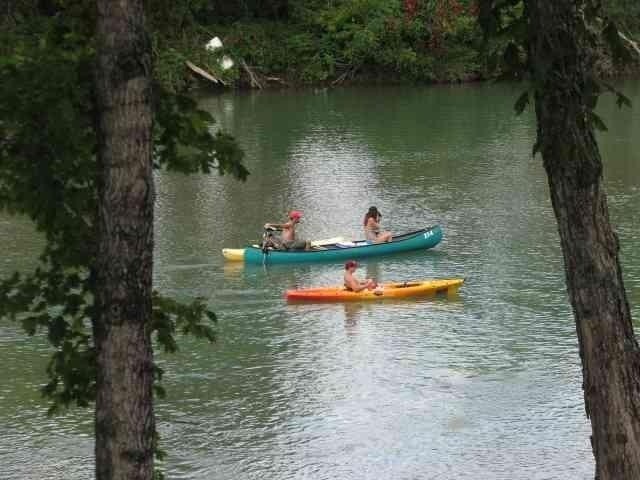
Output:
top-left (221, 224), bottom-right (443, 265)
top-left (286, 277), bottom-right (465, 303)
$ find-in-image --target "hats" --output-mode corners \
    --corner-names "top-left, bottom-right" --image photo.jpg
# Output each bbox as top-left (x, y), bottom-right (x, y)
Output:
top-left (290, 211), bottom-right (299, 218)
top-left (346, 262), bottom-right (355, 268)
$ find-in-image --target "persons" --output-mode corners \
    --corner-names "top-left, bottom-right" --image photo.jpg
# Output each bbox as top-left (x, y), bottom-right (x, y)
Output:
top-left (363, 205), bottom-right (393, 244)
top-left (264, 209), bottom-right (311, 252)
top-left (344, 260), bottom-right (380, 293)
